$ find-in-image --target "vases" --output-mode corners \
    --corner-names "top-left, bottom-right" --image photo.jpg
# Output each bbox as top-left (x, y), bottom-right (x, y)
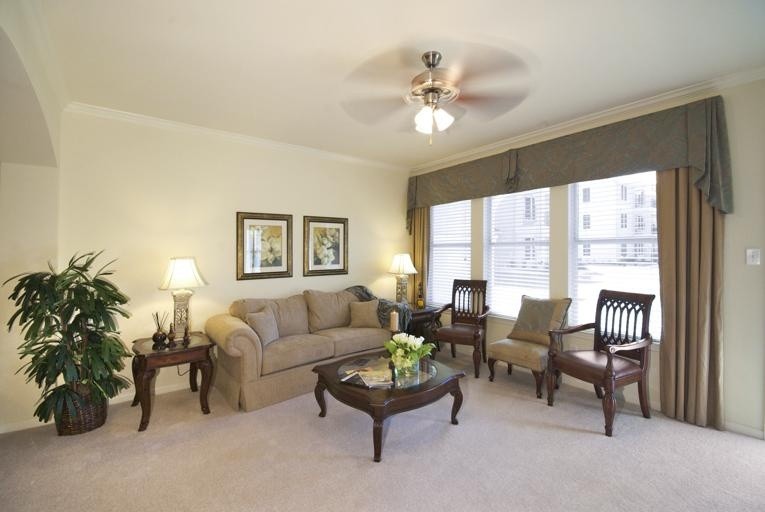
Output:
top-left (394, 358), bottom-right (419, 390)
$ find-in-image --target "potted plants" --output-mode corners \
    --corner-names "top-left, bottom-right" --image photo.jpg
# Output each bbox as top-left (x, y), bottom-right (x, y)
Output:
top-left (0, 249), bottom-right (135, 436)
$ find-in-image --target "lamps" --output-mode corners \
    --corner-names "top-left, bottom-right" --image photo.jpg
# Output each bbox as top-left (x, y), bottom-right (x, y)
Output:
top-left (387, 254), bottom-right (418, 302)
top-left (156, 257), bottom-right (209, 341)
top-left (414, 93), bottom-right (454, 147)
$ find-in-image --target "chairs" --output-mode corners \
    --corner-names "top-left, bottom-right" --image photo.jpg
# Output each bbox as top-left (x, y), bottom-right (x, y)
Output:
top-left (488, 295), bottom-right (572, 398)
top-left (430, 279), bottom-right (492, 378)
top-left (547, 289), bottom-right (655, 436)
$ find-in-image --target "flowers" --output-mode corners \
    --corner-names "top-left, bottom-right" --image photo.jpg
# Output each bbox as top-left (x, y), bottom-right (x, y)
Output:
top-left (384, 333), bottom-right (437, 378)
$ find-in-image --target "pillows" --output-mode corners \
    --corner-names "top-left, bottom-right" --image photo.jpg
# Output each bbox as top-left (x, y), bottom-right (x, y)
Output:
top-left (348, 300), bottom-right (381, 328)
top-left (245, 305), bottom-right (280, 347)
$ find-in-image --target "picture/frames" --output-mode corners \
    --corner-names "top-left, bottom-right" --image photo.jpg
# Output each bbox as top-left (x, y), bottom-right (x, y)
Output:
top-left (303, 216), bottom-right (349, 277)
top-left (236, 212), bottom-right (293, 280)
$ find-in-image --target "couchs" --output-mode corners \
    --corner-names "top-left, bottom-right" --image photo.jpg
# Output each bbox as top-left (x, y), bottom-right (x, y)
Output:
top-left (205, 285), bottom-right (412, 412)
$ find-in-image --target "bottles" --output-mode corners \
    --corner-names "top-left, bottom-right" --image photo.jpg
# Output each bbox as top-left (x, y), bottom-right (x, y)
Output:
top-left (416, 294), bottom-right (426, 309)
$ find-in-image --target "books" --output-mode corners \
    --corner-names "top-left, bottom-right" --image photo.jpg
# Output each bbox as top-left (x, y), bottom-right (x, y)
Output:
top-left (341, 353), bottom-right (392, 391)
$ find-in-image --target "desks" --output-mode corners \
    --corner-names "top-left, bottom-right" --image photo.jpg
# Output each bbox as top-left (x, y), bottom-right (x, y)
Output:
top-left (406, 304), bottom-right (440, 350)
top-left (131, 331), bottom-right (216, 431)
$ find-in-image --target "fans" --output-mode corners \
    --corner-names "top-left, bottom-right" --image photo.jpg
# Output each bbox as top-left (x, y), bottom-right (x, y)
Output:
top-left (340, 38), bottom-right (530, 126)
